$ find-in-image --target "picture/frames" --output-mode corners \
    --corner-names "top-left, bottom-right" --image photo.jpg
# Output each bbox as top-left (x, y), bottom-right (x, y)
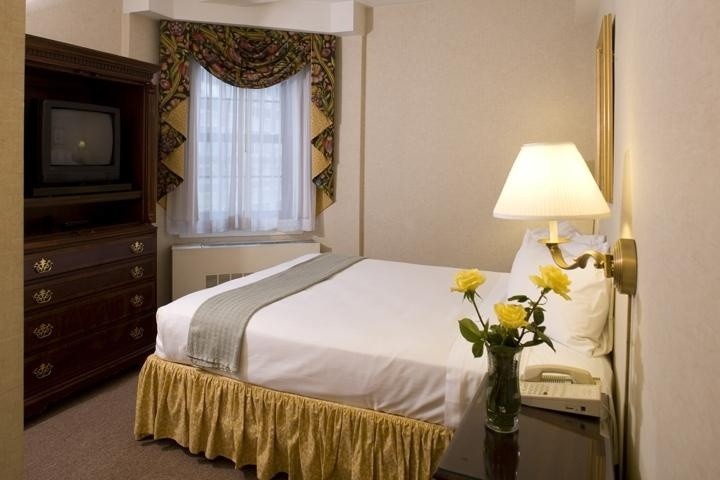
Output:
top-left (595, 13), bottom-right (615, 205)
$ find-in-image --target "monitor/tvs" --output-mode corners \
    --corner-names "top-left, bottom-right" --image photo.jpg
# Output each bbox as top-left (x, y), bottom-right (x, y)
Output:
top-left (37, 98), bottom-right (120, 187)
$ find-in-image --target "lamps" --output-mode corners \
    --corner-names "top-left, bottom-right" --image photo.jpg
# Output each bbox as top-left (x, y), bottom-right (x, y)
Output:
top-left (492, 141), bottom-right (637, 297)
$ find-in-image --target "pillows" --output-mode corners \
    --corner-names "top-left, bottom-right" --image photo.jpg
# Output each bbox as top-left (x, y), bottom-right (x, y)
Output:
top-left (503, 224), bottom-right (617, 360)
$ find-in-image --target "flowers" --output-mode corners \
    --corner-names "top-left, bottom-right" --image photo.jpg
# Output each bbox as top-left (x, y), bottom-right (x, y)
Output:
top-left (450, 264), bottom-right (575, 430)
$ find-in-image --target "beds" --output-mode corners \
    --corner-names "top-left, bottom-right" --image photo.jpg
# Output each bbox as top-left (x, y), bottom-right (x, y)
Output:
top-left (131, 251), bottom-right (616, 480)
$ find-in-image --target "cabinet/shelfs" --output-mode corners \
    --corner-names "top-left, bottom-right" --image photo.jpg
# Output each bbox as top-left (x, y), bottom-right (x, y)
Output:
top-left (23, 223), bottom-right (158, 420)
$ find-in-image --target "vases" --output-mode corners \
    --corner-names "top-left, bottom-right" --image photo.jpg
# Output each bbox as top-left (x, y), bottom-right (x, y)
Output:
top-left (482, 338), bottom-right (523, 434)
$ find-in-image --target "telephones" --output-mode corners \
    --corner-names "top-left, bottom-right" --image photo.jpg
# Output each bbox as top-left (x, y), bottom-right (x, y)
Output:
top-left (519, 364), bottom-right (601, 417)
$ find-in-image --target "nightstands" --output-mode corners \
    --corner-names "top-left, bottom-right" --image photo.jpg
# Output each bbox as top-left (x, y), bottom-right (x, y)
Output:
top-left (431, 371), bottom-right (616, 480)
